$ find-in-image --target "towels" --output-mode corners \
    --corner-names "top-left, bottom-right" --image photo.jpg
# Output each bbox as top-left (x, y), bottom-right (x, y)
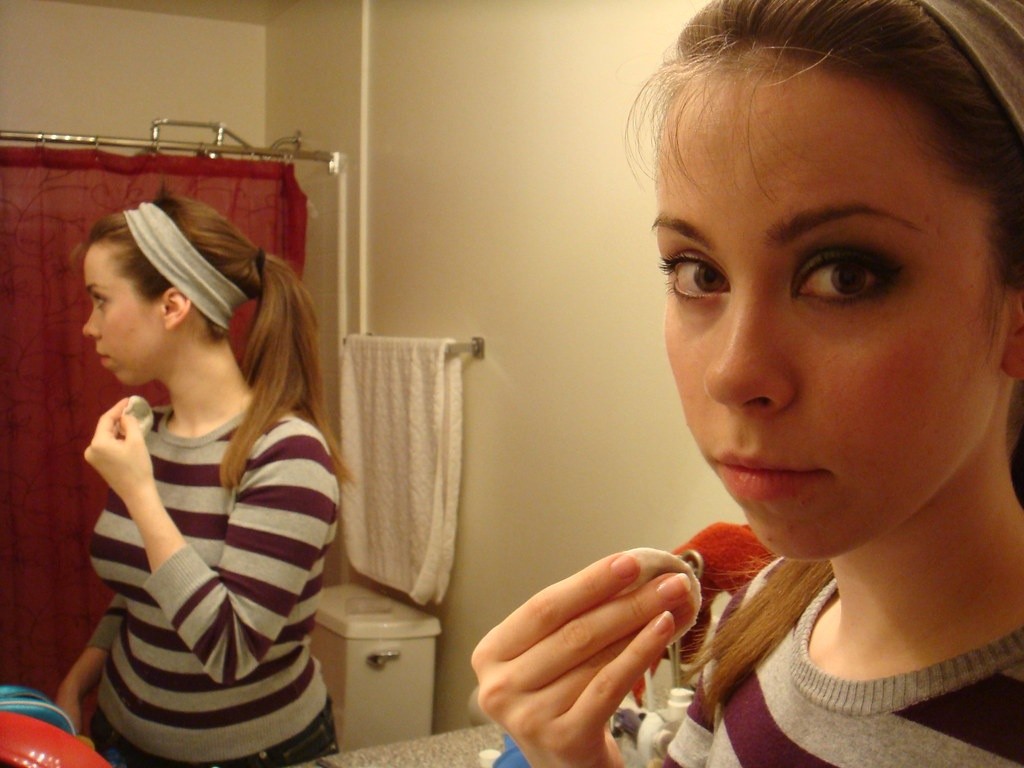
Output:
top-left (339, 334), bottom-right (462, 606)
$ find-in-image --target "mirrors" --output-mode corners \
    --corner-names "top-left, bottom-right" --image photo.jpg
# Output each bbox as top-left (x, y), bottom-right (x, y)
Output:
top-left (1, 1), bottom-right (768, 768)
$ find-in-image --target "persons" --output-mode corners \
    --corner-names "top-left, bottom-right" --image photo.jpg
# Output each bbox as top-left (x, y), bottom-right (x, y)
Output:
top-left (53, 193), bottom-right (352, 768)
top-left (472, 0), bottom-right (1024, 768)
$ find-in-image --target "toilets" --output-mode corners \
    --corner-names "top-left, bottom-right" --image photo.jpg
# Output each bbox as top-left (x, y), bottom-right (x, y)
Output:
top-left (309, 585), bottom-right (441, 753)
